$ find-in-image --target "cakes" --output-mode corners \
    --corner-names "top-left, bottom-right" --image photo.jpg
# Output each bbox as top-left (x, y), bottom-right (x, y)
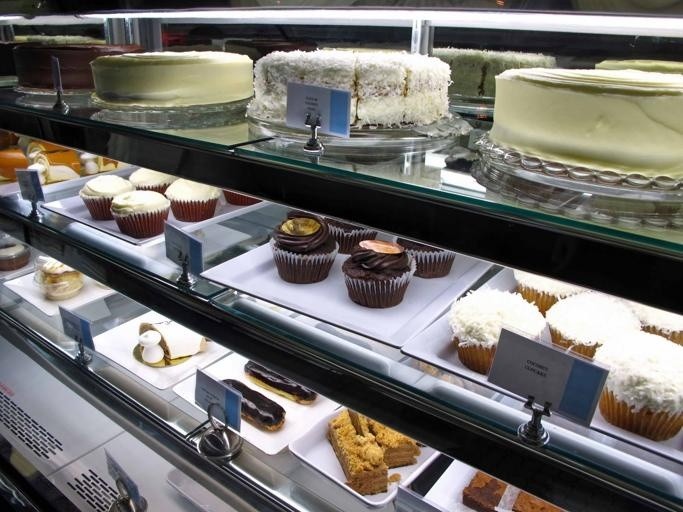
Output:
top-left (632, 304), bottom-right (682, 346)
top-left (369, 419), bottom-right (423, 468)
top-left (86, 50), bottom-right (252, 103)
top-left (110, 190), bottom-right (171, 239)
top-left (15, 41), bottom-right (142, 91)
top-left (79, 174), bottom-right (136, 220)
top-left (595, 54), bottom-right (682, 72)
top-left (397, 239), bottom-right (455, 279)
top-left (0, 40), bottom-right (43, 76)
top-left (329, 413), bottom-right (389, 497)
top-left (251, 48), bottom-right (453, 130)
top-left (165, 178), bottom-right (222, 223)
top-left (272, 213), bottom-right (338, 285)
top-left (327, 219), bottom-right (379, 255)
top-left (448, 286), bottom-right (548, 374)
top-left (517, 269), bottom-right (587, 317)
top-left (129, 166), bottom-right (177, 194)
top-left (226, 38), bottom-right (317, 60)
top-left (342, 239), bottom-right (418, 309)
top-left (462, 471), bottom-right (562, 512)
top-left (491, 66), bottom-right (682, 174)
top-left (225, 191), bottom-right (259, 206)
top-left (594, 334), bottom-right (682, 442)
top-left (543, 293), bottom-right (645, 358)
top-left (432, 48), bottom-right (555, 105)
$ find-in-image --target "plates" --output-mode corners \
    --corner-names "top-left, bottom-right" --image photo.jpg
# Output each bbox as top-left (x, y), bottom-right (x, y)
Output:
top-left (40, 195), bottom-right (269, 247)
top-left (423, 459), bottom-right (558, 512)
top-left (171, 350), bottom-right (342, 455)
top-left (402, 269), bottom-right (683, 465)
top-left (199, 237), bottom-right (496, 347)
top-left (91, 310), bottom-right (231, 389)
top-left (290, 407), bottom-right (437, 508)
top-left (3, 266), bottom-right (118, 317)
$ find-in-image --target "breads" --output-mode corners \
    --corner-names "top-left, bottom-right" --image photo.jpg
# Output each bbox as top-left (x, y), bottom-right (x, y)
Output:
top-left (0, 239), bottom-right (30, 271)
top-left (246, 360), bottom-right (316, 405)
top-left (0, 131), bottom-right (125, 184)
top-left (134, 319), bottom-right (208, 367)
top-left (223, 378), bottom-right (285, 433)
top-left (37, 256), bottom-right (86, 301)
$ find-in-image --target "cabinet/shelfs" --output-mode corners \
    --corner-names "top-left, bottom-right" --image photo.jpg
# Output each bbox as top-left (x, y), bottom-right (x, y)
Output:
top-left (0, 8), bottom-right (682, 512)
